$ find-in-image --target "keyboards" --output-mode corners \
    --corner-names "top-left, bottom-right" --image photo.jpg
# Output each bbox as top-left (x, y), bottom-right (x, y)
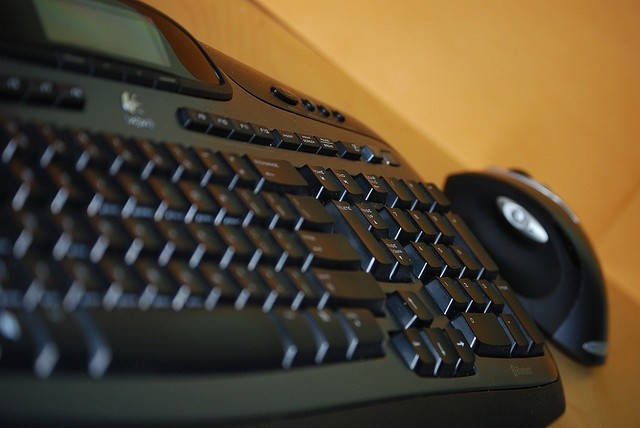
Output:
top-left (1, 1), bottom-right (566, 427)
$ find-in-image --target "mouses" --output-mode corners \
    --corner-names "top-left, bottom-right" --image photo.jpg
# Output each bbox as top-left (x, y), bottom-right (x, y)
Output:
top-left (444, 166), bottom-right (609, 366)
